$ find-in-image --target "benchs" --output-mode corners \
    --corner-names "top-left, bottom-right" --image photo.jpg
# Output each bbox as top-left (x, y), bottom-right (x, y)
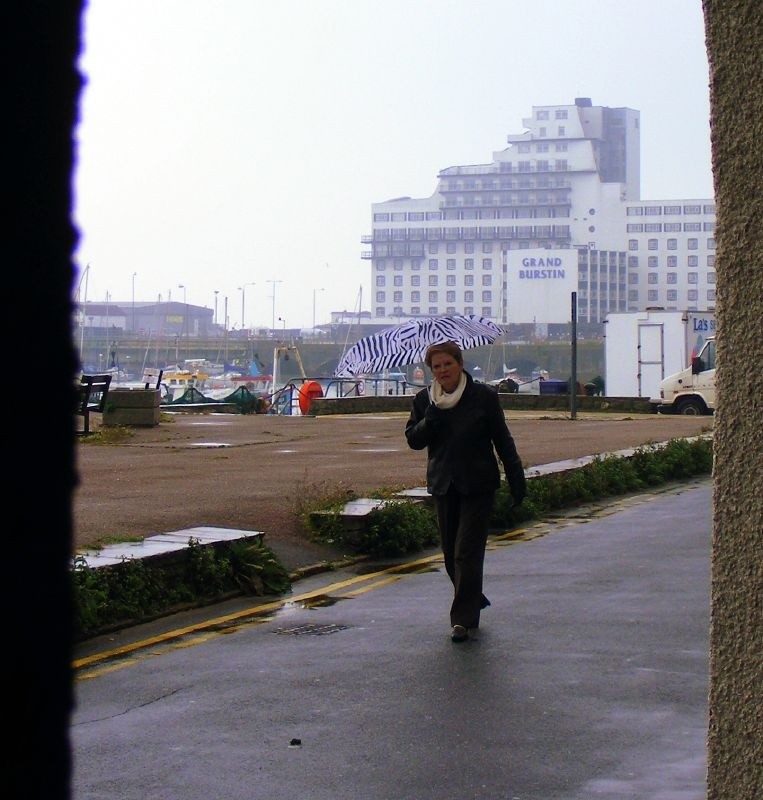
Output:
top-left (73, 374), bottom-right (113, 436)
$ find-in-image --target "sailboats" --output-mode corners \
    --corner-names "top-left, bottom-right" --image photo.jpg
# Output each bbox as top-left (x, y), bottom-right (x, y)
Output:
top-left (72, 263), bottom-right (533, 415)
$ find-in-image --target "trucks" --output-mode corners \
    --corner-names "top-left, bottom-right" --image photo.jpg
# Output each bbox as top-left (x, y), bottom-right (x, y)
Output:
top-left (601, 305), bottom-right (717, 403)
top-left (649, 336), bottom-right (718, 416)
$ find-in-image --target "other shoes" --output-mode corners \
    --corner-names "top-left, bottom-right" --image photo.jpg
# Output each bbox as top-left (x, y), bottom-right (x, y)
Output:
top-left (451, 624), bottom-right (471, 643)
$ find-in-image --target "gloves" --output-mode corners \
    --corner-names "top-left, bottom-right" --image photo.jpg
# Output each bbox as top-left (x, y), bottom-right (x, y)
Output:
top-left (424, 401), bottom-right (448, 432)
top-left (507, 486), bottom-right (525, 509)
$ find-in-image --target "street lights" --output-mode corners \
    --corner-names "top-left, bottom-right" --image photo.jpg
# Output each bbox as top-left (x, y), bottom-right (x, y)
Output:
top-left (132, 271), bottom-right (138, 332)
top-left (278, 317), bottom-right (287, 329)
top-left (312, 287), bottom-right (325, 343)
top-left (179, 285), bottom-right (187, 325)
top-left (214, 291), bottom-right (219, 325)
top-left (237, 282), bottom-right (258, 329)
top-left (265, 278), bottom-right (284, 339)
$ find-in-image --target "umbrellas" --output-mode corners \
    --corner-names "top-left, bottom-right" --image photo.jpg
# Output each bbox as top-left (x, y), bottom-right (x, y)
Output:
top-left (332, 314), bottom-right (509, 405)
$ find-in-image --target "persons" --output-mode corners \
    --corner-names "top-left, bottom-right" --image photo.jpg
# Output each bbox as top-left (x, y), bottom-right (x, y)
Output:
top-left (406, 341), bottom-right (528, 641)
